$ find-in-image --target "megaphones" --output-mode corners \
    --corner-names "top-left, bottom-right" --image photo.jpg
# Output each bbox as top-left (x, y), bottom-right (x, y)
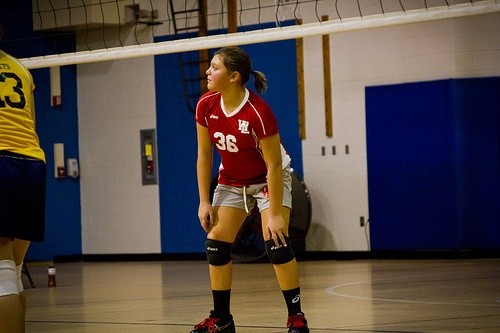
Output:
top-left (124, 3), bottom-right (151, 21)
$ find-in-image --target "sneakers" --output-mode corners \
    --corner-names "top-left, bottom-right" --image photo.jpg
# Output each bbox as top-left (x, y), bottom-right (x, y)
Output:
top-left (190, 310), bottom-right (235, 333)
top-left (287, 310), bottom-right (309, 333)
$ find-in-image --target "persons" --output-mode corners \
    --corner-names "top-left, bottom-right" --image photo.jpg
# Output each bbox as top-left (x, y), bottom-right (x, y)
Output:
top-left (191, 46), bottom-right (309, 333)
top-left (287, 172), bottom-right (309, 240)
top-left (0, 48), bottom-right (47, 333)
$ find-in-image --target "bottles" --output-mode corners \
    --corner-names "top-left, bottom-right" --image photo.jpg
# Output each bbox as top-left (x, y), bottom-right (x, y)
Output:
top-left (47, 260), bottom-right (57, 288)
